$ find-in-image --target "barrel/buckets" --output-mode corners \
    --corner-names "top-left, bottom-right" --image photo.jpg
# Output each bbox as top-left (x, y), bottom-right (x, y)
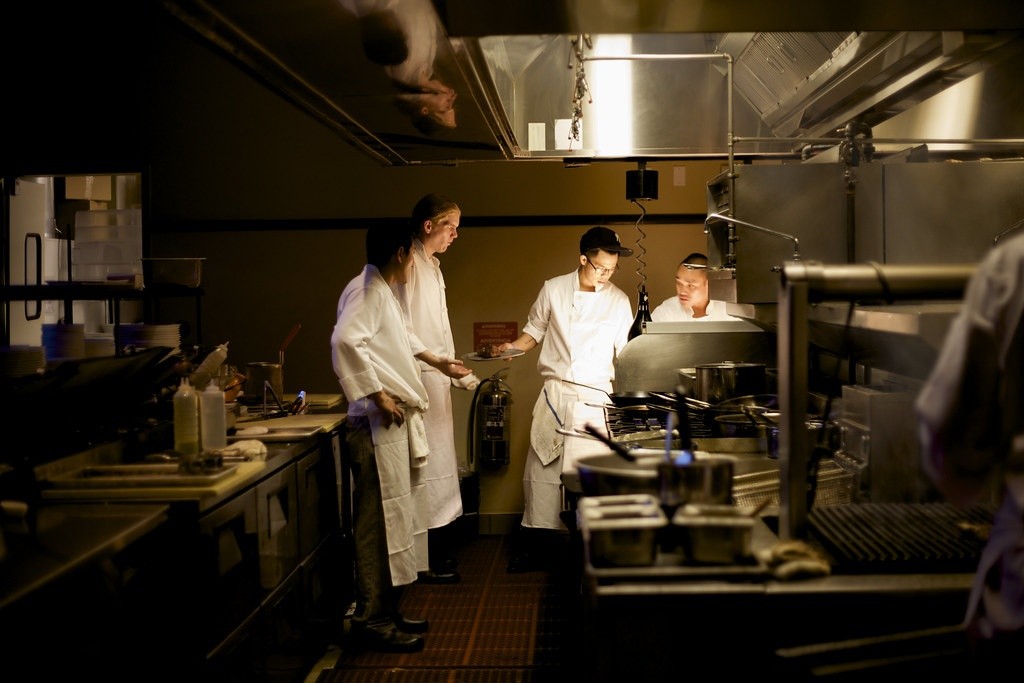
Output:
top-left (694, 359), bottom-right (767, 404)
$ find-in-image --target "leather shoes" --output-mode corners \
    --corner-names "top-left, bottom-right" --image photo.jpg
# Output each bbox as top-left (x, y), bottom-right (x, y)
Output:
top-left (417, 564), bottom-right (460, 583)
top-left (351, 627), bottom-right (423, 652)
top-left (394, 613), bottom-right (429, 633)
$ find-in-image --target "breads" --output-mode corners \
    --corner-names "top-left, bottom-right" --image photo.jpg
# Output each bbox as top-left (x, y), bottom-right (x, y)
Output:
top-left (476, 342), bottom-right (502, 358)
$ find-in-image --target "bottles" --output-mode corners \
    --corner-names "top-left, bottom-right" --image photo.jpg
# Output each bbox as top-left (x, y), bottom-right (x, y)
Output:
top-left (627, 283), bottom-right (653, 342)
top-left (173, 340), bottom-right (229, 455)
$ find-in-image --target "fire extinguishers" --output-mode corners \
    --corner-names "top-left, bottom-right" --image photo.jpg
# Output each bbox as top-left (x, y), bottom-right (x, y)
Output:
top-left (470, 368), bottom-right (512, 465)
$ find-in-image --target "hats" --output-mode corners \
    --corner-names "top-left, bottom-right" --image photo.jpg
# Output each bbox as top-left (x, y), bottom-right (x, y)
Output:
top-left (580, 226), bottom-right (633, 256)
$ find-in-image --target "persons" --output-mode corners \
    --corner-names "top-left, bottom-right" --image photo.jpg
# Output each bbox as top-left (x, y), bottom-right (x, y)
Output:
top-left (330, 214), bottom-right (431, 653)
top-left (916, 234), bottom-right (1023, 683)
top-left (387, 194), bottom-right (474, 583)
top-left (346, 0), bottom-right (460, 137)
top-left (497, 226), bottom-right (634, 575)
top-left (647, 253), bottom-right (746, 322)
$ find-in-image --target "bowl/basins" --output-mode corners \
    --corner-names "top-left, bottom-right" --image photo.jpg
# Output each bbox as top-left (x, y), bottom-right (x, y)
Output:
top-left (573, 448), bottom-right (734, 515)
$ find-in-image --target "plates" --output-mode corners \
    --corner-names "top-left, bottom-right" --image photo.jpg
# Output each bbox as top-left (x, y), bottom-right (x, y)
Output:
top-left (226, 427), bottom-right (323, 437)
top-left (44, 464), bottom-right (242, 484)
top-left (7, 322), bottom-right (182, 376)
top-left (461, 349), bottom-right (525, 361)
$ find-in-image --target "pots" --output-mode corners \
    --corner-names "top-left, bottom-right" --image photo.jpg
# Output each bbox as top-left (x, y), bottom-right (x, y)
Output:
top-left (553, 377), bottom-right (838, 459)
